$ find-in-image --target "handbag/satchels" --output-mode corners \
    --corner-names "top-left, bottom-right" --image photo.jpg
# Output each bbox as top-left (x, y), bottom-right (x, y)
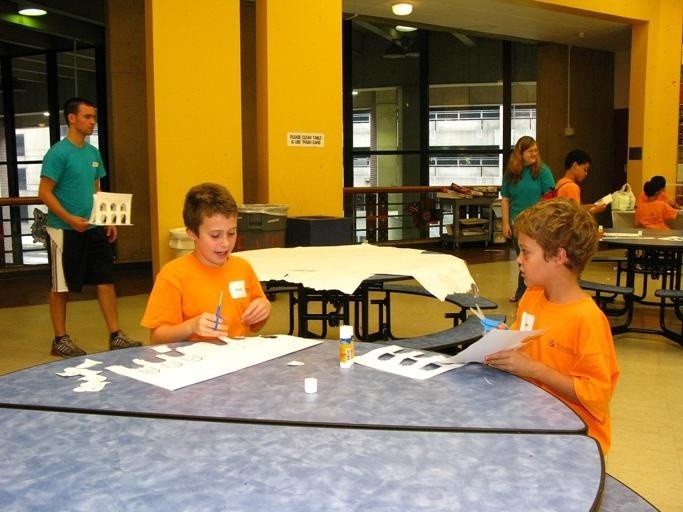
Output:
top-left (610, 183), bottom-right (636, 211)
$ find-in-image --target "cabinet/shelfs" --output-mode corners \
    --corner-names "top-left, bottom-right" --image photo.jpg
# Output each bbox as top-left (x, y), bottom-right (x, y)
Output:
top-left (440, 198), bottom-right (495, 248)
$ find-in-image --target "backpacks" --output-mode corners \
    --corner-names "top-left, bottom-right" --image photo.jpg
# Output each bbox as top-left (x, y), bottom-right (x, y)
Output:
top-left (540, 190), bottom-right (558, 201)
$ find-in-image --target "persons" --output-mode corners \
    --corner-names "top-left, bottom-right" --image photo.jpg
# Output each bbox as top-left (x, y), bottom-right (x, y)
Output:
top-left (484, 198), bottom-right (619, 457)
top-left (554, 148), bottom-right (606, 215)
top-left (634, 176), bottom-right (683, 209)
top-left (39, 97), bottom-right (143, 359)
top-left (140, 183), bottom-right (271, 344)
top-left (634, 182), bottom-right (682, 229)
top-left (501, 135), bottom-right (554, 301)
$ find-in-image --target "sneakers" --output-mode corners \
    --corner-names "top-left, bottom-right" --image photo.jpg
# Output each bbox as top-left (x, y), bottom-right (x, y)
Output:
top-left (50, 334), bottom-right (87, 359)
top-left (109, 330), bottom-right (143, 351)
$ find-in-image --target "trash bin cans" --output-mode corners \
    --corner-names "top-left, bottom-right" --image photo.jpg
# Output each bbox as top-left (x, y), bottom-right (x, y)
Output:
top-left (229, 203), bottom-right (288, 253)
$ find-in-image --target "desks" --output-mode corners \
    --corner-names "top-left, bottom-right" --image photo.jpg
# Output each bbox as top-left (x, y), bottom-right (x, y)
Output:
top-left (0, 336), bottom-right (606, 511)
top-left (231, 246), bottom-right (480, 343)
top-left (598, 227), bottom-right (683, 345)
top-left (611, 210), bottom-right (683, 231)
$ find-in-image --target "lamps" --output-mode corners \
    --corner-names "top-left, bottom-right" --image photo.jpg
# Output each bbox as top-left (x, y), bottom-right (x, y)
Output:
top-left (391, 2), bottom-right (413, 16)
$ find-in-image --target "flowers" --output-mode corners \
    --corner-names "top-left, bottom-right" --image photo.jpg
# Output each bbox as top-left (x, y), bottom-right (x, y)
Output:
top-left (408, 198), bottom-right (444, 231)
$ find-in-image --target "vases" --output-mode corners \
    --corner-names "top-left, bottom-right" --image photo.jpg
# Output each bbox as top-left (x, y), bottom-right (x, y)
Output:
top-left (419, 223), bottom-right (429, 240)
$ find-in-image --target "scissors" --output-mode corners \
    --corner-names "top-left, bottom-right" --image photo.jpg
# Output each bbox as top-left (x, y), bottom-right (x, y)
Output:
top-left (469, 303), bottom-right (510, 340)
top-left (214, 291), bottom-right (223, 331)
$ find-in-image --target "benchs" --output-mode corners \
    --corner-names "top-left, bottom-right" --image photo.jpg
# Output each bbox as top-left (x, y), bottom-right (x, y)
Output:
top-left (655, 288), bottom-right (683, 334)
top-left (381, 315), bottom-right (507, 348)
top-left (578, 256), bottom-right (634, 333)
top-left (596, 473), bottom-right (660, 512)
top-left (263, 285), bottom-right (496, 335)
